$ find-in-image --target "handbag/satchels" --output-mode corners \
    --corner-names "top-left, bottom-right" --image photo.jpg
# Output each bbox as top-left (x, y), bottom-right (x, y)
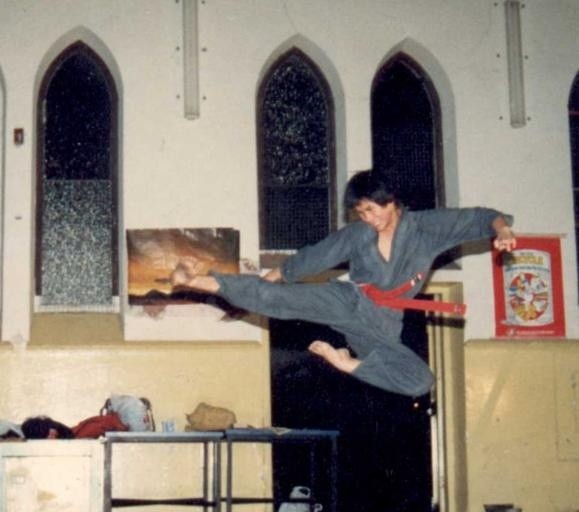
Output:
top-left (22, 395), bottom-right (156, 439)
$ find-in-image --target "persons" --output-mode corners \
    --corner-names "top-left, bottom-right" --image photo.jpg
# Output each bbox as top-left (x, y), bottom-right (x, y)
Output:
top-left (172, 170), bottom-right (516, 399)
